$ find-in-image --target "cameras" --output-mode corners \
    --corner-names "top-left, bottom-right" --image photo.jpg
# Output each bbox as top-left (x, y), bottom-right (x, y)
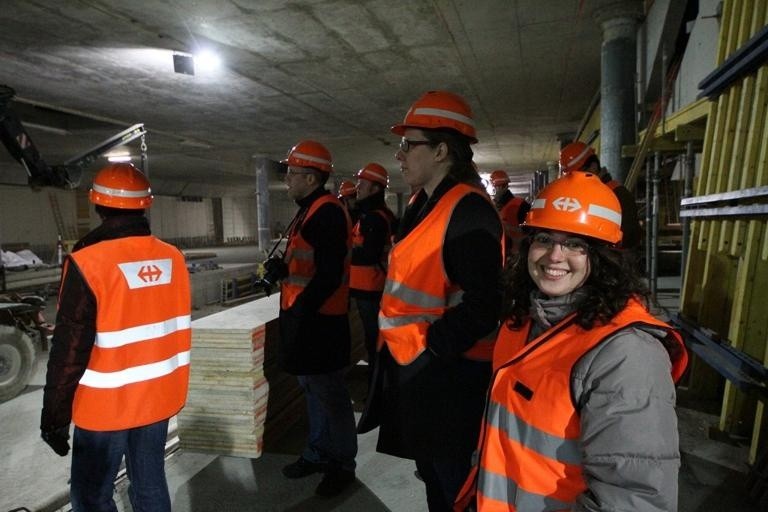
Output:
top-left (254, 253), bottom-right (289, 297)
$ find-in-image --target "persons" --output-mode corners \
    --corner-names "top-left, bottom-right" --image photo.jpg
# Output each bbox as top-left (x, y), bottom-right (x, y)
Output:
top-left (490, 170), bottom-right (531, 254)
top-left (559, 141), bottom-right (642, 251)
top-left (41, 163), bottom-right (192, 512)
top-left (452, 172), bottom-right (688, 512)
top-left (349, 163), bottom-right (396, 402)
top-left (279, 140), bottom-right (357, 498)
top-left (356, 91), bottom-right (506, 512)
top-left (337, 180), bottom-right (357, 203)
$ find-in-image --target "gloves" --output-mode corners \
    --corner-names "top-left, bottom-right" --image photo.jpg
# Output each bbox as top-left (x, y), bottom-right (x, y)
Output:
top-left (39, 428), bottom-right (70, 457)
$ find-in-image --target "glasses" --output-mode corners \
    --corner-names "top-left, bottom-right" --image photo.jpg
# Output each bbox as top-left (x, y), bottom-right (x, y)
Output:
top-left (398, 136), bottom-right (434, 153)
top-left (529, 232), bottom-right (589, 253)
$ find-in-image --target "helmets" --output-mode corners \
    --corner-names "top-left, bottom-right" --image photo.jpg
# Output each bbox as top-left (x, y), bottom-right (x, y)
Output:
top-left (390, 89), bottom-right (478, 146)
top-left (280, 134), bottom-right (388, 198)
top-left (516, 138), bottom-right (624, 250)
top-left (86, 160), bottom-right (157, 213)
top-left (489, 168), bottom-right (511, 186)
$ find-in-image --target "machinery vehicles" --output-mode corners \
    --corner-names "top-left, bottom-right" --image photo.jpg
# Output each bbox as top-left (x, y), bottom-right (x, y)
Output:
top-left (0, 81), bottom-right (153, 407)
